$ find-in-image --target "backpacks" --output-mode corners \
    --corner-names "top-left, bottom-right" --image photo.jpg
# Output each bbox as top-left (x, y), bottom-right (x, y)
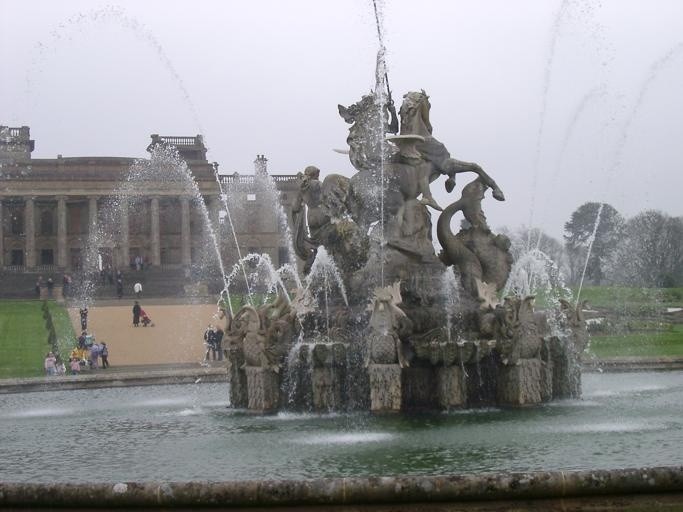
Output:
top-left (102, 348), bottom-right (108, 357)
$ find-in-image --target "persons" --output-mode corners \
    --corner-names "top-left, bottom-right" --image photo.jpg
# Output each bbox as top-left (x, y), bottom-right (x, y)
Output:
top-left (290, 164), bottom-right (322, 213)
top-left (203, 324), bottom-right (216, 360)
top-left (214, 325), bottom-right (223, 360)
top-left (79, 306), bottom-right (88, 332)
top-left (347, 47), bottom-right (400, 161)
top-left (70, 331), bottom-right (109, 375)
top-left (33, 255), bottom-right (149, 300)
top-left (44, 351), bottom-right (55, 376)
top-left (132, 301), bottom-right (140, 327)
top-left (55, 362), bottom-right (66, 374)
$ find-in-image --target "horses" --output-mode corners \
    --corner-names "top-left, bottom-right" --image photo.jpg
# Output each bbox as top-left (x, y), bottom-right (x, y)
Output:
top-left (320, 86), bottom-right (506, 227)
